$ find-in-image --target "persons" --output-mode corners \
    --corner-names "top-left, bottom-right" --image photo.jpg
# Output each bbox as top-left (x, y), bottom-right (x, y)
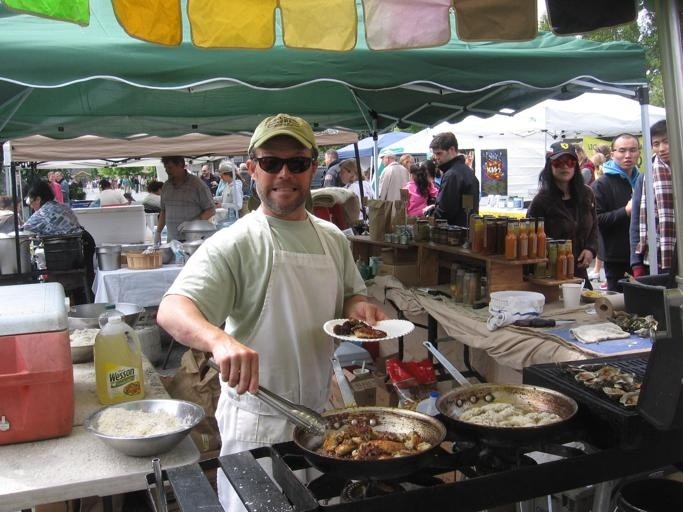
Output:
top-left (55, 171), bottom-right (70, 203)
top-left (98, 179), bottom-right (128, 206)
top-left (574, 144), bottom-right (599, 187)
top-left (400, 154), bottom-right (414, 170)
top-left (590, 132), bottom-right (641, 293)
top-left (153, 155), bottom-right (215, 244)
top-left (379, 151), bottom-right (409, 223)
top-left (0, 194), bottom-right (24, 233)
top-left (324, 149), bottom-right (341, 187)
top-left (402, 163), bottom-right (439, 220)
top-left (200, 164), bottom-right (220, 196)
top-left (142, 180), bottom-right (164, 213)
top-left (156, 113), bottom-right (390, 512)
top-left (19, 182), bottom-right (95, 276)
top-left (339, 157), bottom-right (375, 222)
top-left (421, 131), bottom-right (479, 226)
top-left (213, 160), bottom-right (243, 214)
top-left (525, 141), bottom-right (600, 292)
top-left (47, 172), bottom-right (64, 204)
top-left (630, 119), bottom-right (678, 277)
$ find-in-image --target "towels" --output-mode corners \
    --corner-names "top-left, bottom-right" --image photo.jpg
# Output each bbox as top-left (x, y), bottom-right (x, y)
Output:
top-left (486, 295), bottom-right (540, 333)
top-left (570, 321), bottom-right (630, 344)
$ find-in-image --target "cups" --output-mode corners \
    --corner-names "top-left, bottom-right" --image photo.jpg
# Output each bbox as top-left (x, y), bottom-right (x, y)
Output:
top-left (561, 283), bottom-right (582, 308)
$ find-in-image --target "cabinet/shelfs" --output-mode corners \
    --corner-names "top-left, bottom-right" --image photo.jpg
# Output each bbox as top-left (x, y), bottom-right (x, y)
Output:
top-left (346, 232), bottom-right (584, 301)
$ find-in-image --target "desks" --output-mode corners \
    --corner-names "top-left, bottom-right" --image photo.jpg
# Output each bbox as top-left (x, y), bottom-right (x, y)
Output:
top-left (1, 323), bottom-right (202, 511)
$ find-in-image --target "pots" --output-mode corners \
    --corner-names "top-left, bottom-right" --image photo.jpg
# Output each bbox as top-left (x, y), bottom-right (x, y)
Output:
top-left (421, 341), bottom-right (579, 441)
top-left (291, 356), bottom-right (445, 478)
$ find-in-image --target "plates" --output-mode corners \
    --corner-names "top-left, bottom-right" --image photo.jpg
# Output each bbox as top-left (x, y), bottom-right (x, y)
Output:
top-left (321, 316), bottom-right (415, 343)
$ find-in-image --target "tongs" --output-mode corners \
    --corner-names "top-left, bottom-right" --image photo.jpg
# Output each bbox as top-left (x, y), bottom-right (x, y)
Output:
top-left (206, 359), bottom-right (332, 436)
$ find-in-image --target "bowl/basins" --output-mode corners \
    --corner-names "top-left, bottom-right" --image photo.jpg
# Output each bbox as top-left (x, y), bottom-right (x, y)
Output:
top-left (67, 304), bottom-right (145, 332)
top-left (69, 328), bottom-right (100, 365)
top-left (85, 399), bottom-right (205, 456)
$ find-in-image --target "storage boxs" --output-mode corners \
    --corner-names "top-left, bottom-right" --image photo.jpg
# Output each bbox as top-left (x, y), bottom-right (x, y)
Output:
top-left (0, 283), bottom-right (74, 445)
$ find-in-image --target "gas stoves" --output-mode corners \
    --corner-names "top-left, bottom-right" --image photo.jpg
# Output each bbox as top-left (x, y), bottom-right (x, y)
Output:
top-left (271, 411), bottom-right (650, 511)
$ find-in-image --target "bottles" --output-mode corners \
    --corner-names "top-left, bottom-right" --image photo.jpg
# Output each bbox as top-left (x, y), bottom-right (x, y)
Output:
top-left (415, 220), bottom-right (430, 241)
top-left (496, 217), bottom-right (505, 255)
top-left (566, 240), bottom-right (574, 279)
top-left (518, 219), bottom-right (528, 259)
top-left (434, 219), bottom-right (448, 228)
top-left (534, 262), bottom-right (546, 280)
top-left (450, 264), bottom-right (461, 297)
top-left (528, 218), bottom-right (537, 259)
top-left (537, 217), bottom-right (546, 258)
top-left (484, 218), bottom-right (496, 255)
top-left (472, 216), bottom-right (484, 252)
top-left (548, 240), bottom-right (557, 280)
top-left (462, 270), bottom-right (481, 306)
top-left (505, 220), bottom-right (517, 260)
top-left (557, 241), bottom-right (568, 281)
top-left (455, 268), bottom-right (468, 303)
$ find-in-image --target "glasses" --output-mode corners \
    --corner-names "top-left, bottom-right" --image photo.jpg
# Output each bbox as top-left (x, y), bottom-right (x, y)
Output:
top-left (253, 156), bottom-right (316, 174)
top-left (550, 158), bottom-right (576, 169)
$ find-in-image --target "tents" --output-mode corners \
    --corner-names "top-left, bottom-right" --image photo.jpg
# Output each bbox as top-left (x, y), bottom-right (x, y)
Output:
top-left (6, 128), bottom-right (366, 275)
top-left (336, 130), bottom-right (414, 181)
top-left (0, 0), bottom-right (659, 277)
top-left (380, 89), bottom-right (669, 202)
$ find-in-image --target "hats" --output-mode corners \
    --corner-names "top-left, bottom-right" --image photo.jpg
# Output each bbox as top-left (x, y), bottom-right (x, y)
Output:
top-left (247, 112), bottom-right (319, 159)
top-left (546, 141), bottom-right (579, 160)
top-left (219, 161), bottom-right (237, 174)
top-left (378, 151), bottom-right (396, 159)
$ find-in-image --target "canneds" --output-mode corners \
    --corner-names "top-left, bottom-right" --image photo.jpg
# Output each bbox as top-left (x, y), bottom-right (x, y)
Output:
top-left (430, 228), bottom-right (439, 243)
top-left (439, 227), bottom-right (448, 245)
top-left (448, 228), bottom-right (465, 246)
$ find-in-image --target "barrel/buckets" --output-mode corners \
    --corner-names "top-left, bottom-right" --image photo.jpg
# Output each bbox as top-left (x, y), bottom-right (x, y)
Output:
top-left (95, 244), bottom-right (122, 271)
top-left (94, 316), bottom-right (144, 404)
